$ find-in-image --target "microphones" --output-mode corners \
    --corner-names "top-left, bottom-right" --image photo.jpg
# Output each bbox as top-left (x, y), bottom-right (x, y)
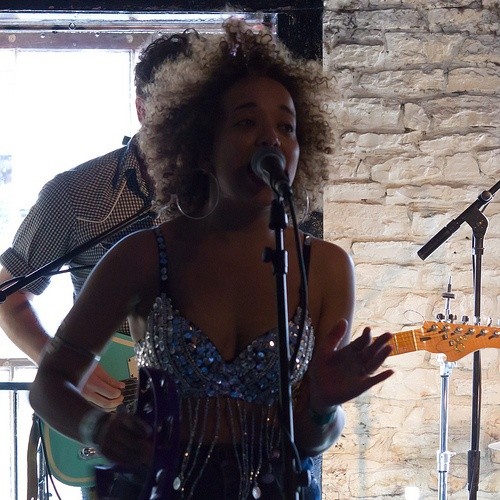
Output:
top-left (251, 146), bottom-right (293, 198)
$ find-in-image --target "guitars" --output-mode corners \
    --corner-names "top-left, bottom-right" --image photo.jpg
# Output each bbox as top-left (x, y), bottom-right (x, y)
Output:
top-left (39, 313), bottom-right (500, 487)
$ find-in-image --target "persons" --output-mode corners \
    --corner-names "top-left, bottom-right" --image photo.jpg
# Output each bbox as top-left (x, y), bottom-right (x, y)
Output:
top-left (0, 30), bottom-right (210, 500)
top-left (29, 9), bottom-right (393, 500)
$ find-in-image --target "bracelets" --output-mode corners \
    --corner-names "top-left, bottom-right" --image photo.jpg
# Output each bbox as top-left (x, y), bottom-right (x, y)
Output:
top-left (306, 402), bottom-right (335, 425)
top-left (91, 410), bottom-right (112, 445)
